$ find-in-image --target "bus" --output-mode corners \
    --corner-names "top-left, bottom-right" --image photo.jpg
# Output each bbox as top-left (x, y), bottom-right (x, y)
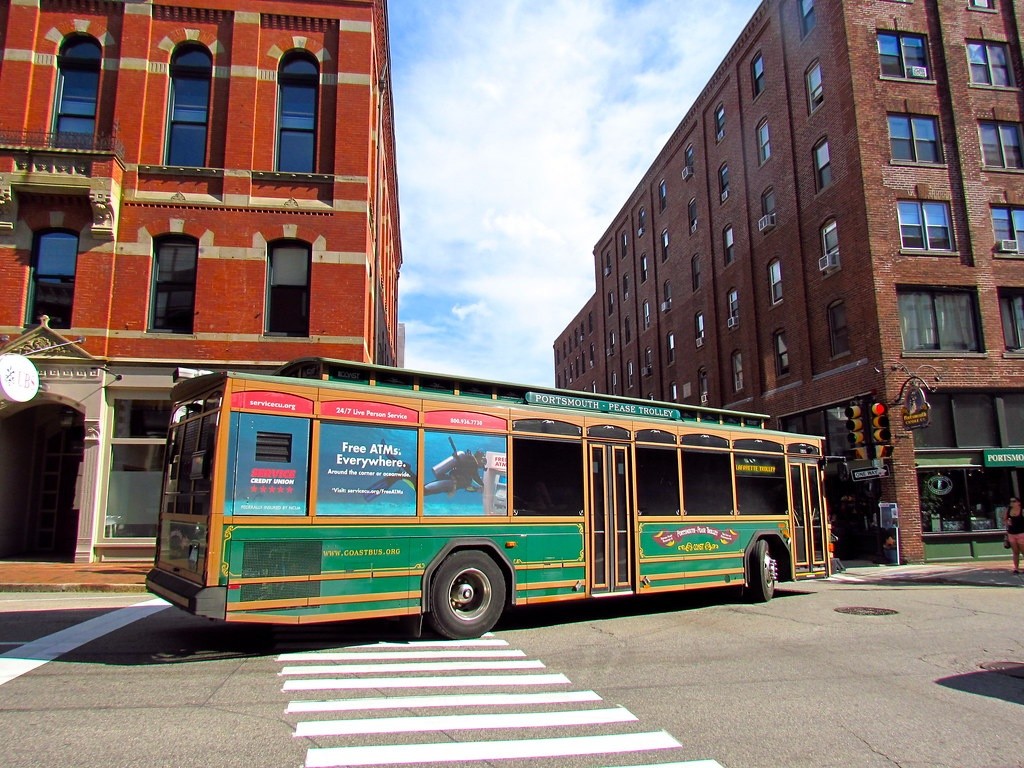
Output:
top-left (143, 356), bottom-right (847, 641)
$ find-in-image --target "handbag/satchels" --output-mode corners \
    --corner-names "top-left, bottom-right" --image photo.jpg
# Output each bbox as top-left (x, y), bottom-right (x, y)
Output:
top-left (1004, 535), bottom-right (1011, 548)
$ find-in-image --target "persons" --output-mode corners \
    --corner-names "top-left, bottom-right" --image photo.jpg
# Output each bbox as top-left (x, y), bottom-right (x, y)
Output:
top-left (363, 437), bottom-right (491, 503)
top-left (1001, 496), bottom-right (1024, 574)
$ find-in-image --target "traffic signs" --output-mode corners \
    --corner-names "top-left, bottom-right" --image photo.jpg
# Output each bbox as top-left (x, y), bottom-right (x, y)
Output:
top-left (851, 465), bottom-right (890, 481)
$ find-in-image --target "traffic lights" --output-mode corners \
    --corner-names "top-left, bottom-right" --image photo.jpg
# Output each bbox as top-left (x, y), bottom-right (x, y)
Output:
top-left (845, 405), bottom-right (866, 443)
top-left (869, 402), bottom-right (891, 444)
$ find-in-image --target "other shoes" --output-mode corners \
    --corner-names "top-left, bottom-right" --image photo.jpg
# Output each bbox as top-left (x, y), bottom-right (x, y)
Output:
top-left (1013, 570), bottom-right (1019, 575)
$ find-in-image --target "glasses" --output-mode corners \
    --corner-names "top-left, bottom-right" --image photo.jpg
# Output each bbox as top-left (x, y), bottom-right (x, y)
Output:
top-left (1010, 501), bottom-right (1016, 503)
